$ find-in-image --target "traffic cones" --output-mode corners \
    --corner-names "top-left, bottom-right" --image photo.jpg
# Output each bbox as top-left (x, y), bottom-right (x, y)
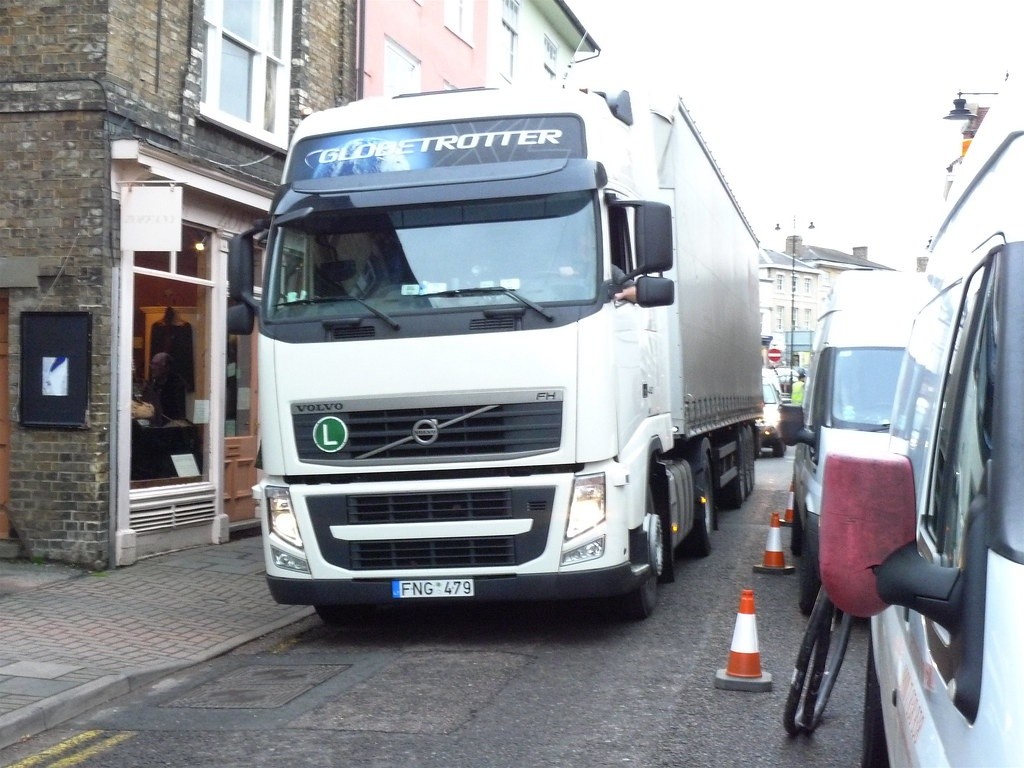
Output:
top-left (715, 590), bottom-right (773, 693)
top-left (753, 512), bottom-right (797, 575)
top-left (779, 481), bottom-right (795, 527)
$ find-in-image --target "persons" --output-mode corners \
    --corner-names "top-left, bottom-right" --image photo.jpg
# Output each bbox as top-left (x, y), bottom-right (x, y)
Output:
top-left (790, 375), bottom-right (806, 405)
top-left (149, 306), bottom-right (194, 392)
top-left (132, 353), bottom-right (185, 481)
top-left (558, 231), bottom-right (638, 304)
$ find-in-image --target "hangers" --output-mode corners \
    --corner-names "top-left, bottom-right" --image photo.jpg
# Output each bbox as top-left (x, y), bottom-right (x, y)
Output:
top-left (157, 308), bottom-right (186, 327)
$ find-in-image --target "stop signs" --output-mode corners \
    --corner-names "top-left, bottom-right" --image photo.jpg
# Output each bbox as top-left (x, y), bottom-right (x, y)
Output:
top-left (768, 348), bottom-right (782, 362)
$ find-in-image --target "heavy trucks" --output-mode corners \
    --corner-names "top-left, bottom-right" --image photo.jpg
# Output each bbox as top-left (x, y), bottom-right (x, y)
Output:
top-left (227, 84), bottom-right (764, 630)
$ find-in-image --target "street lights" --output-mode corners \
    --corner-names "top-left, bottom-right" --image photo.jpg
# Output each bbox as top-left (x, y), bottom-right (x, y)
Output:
top-left (775, 216), bottom-right (816, 399)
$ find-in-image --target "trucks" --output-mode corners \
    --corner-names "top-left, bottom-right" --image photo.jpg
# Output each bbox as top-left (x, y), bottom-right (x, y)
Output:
top-left (780, 269), bottom-right (925, 616)
top-left (818, 75), bottom-right (1024, 767)
top-left (755, 368), bottom-right (800, 458)
